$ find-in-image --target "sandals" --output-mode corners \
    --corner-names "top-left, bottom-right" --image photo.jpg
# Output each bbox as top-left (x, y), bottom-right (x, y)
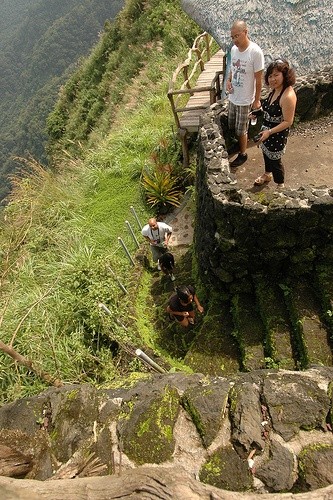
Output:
top-left (255, 174), bottom-right (272, 185)
top-left (277, 184), bottom-right (284, 189)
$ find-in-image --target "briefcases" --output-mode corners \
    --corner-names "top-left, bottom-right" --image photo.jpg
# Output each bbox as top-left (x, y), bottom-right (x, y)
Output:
top-left (158, 252), bottom-right (174, 274)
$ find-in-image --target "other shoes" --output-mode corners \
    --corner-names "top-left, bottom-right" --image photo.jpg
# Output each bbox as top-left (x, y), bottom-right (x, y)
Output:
top-left (227, 140), bottom-right (241, 154)
top-left (230, 153), bottom-right (247, 167)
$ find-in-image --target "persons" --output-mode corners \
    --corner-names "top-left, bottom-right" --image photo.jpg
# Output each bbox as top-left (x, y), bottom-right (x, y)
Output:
top-left (249, 60), bottom-right (297, 189)
top-left (167, 285), bottom-right (205, 331)
top-left (226, 19), bottom-right (265, 167)
top-left (141, 218), bottom-right (175, 281)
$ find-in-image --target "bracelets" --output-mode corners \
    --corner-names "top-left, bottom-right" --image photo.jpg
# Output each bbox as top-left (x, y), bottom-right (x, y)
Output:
top-left (269, 129), bottom-right (273, 135)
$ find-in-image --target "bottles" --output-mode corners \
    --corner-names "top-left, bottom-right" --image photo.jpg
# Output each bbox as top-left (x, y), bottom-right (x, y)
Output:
top-left (251, 118), bottom-right (257, 125)
